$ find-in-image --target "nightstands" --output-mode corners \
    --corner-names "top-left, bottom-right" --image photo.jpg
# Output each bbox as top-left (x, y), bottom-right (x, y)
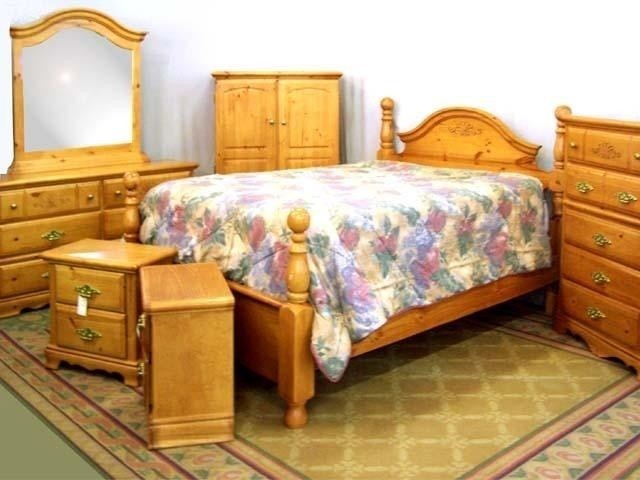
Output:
top-left (40, 237), bottom-right (179, 389)
top-left (140, 261), bottom-right (235, 447)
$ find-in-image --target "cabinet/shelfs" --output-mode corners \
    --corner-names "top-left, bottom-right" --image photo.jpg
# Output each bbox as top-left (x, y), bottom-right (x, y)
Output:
top-left (558, 115), bottom-right (640, 377)
top-left (0, 162), bottom-right (199, 319)
top-left (211, 70), bottom-right (344, 174)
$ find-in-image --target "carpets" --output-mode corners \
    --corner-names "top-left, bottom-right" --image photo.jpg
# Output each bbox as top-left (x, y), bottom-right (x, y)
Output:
top-left (0, 301), bottom-right (640, 480)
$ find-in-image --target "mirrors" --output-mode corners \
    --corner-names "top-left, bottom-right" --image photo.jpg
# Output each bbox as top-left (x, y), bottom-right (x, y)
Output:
top-left (6, 8), bottom-right (148, 176)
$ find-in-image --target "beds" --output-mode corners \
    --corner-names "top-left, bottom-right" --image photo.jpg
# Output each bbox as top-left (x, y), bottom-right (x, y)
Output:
top-left (124, 95), bottom-right (572, 431)
